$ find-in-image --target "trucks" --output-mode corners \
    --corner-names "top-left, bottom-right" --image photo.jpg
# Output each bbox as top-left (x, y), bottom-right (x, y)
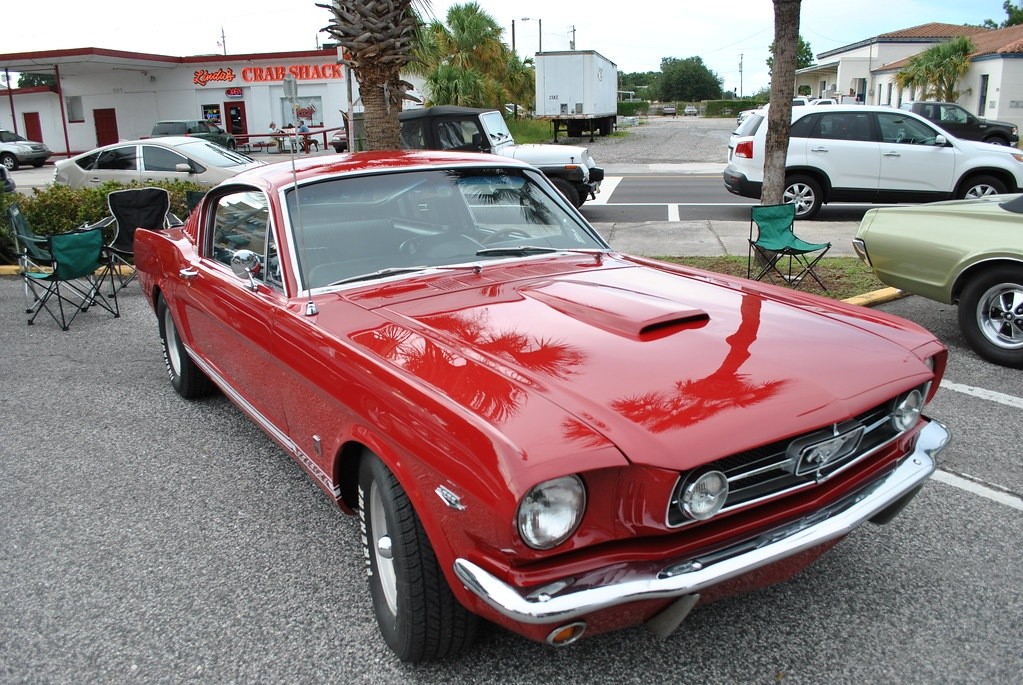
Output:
top-left (534, 51), bottom-right (617, 136)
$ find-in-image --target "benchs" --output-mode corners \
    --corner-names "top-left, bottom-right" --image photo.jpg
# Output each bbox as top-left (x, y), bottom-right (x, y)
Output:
top-left (253, 143), bottom-right (276, 153)
top-left (243, 141), bottom-right (264, 154)
top-left (291, 138), bottom-right (310, 151)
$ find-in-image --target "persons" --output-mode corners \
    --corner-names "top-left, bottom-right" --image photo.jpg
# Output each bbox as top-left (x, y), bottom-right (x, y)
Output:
top-left (297, 120), bottom-right (311, 151)
top-left (268, 122), bottom-right (281, 153)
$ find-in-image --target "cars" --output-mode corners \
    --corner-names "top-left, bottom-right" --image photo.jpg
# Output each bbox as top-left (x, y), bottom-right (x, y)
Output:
top-left (853, 194), bottom-right (1023, 368)
top-left (154, 119), bottom-right (236, 152)
top-left (722, 104), bottom-right (1023, 220)
top-left (135, 149), bottom-right (947, 664)
top-left (684, 106), bottom-right (697, 116)
top-left (346, 107), bottom-right (603, 213)
top-left (0, 130), bottom-right (50, 171)
top-left (53, 136), bottom-right (269, 193)
top-left (663, 105), bottom-right (676, 116)
top-left (737, 97), bottom-right (811, 126)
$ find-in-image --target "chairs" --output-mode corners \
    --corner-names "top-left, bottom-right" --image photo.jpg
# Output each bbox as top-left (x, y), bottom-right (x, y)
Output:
top-left (103, 187), bottom-right (184, 298)
top-left (19, 227), bottom-right (121, 332)
top-left (748, 203), bottom-right (832, 295)
top-left (8, 202), bottom-right (96, 312)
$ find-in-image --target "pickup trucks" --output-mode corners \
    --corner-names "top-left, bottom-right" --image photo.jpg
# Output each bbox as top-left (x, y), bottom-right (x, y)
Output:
top-left (839, 101), bottom-right (1018, 148)
